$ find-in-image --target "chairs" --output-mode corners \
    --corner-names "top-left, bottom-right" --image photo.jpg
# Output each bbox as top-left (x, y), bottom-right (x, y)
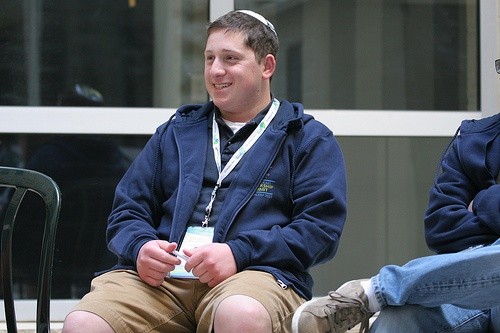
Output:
top-left (0, 166), bottom-right (62, 333)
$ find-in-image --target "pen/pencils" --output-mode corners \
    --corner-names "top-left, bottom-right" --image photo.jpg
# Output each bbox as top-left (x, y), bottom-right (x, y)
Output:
top-left (173, 250), bottom-right (189, 260)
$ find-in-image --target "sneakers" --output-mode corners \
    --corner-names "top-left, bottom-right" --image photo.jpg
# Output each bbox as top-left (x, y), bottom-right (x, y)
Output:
top-left (291, 277), bottom-right (375, 333)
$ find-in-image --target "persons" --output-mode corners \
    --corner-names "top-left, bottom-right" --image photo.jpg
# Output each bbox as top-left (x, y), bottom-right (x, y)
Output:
top-left (61, 10), bottom-right (347, 333)
top-left (291, 58), bottom-right (500, 333)
